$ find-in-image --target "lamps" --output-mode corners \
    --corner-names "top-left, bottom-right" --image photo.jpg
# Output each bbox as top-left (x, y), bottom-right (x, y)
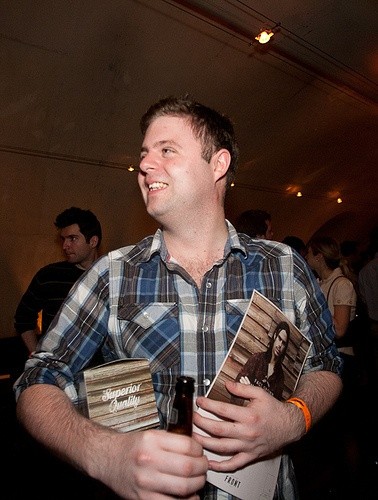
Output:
top-left (248, 19), bottom-right (282, 50)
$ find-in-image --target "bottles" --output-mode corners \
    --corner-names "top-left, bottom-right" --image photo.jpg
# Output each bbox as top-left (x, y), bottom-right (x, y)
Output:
top-left (165, 376), bottom-right (196, 439)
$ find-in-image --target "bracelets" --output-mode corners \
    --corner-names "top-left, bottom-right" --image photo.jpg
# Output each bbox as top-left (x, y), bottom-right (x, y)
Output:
top-left (283, 398), bottom-right (311, 436)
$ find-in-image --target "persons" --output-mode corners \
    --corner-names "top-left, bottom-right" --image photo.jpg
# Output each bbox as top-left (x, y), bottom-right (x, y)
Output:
top-left (228, 322), bottom-right (291, 406)
top-left (15, 206), bottom-right (378, 500)
top-left (12, 97), bottom-right (349, 499)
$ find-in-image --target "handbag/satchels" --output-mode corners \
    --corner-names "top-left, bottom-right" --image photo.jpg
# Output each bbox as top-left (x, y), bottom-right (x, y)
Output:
top-left (327, 275), bottom-right (366, 349)
